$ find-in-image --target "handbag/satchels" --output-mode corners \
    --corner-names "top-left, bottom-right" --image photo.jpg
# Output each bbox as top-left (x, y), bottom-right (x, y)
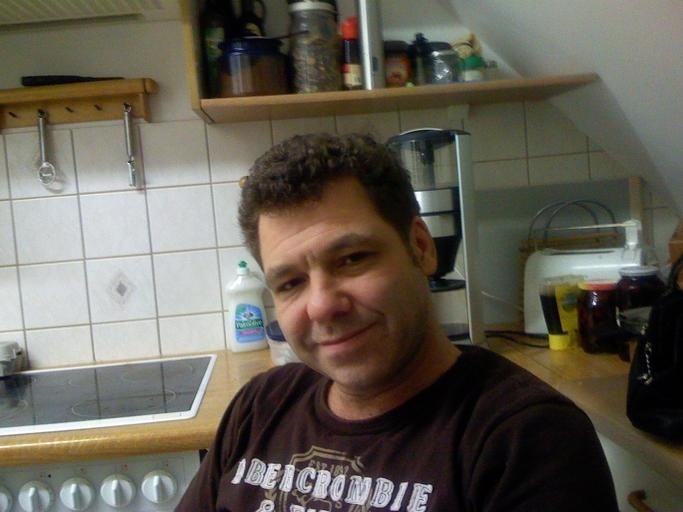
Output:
top-left (625, 255), bottom-right (683, 445)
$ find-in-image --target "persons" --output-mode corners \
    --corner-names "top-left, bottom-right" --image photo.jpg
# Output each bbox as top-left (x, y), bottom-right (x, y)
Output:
top-left (172, 132), bottom-right (620, 512)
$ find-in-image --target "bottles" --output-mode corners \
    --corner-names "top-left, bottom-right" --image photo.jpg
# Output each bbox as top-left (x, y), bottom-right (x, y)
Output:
top-left (214, 37), bottom-right (290, 99)
top-left (616, 267), bottom-right (667, 361)
top-left (287, 0), bottom-right (341, 93)
top-left (577, 280), bottom-right (618, 353)
top-left (421, 41), bottom-right (460, 84)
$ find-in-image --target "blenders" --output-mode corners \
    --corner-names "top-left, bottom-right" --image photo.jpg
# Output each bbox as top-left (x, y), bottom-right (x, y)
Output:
top-left (381, 128), bottom-right (486, 346)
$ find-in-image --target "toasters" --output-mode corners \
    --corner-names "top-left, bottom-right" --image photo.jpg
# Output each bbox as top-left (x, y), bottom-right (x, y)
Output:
top-left (522, 220), bottom-right (646, 335)
